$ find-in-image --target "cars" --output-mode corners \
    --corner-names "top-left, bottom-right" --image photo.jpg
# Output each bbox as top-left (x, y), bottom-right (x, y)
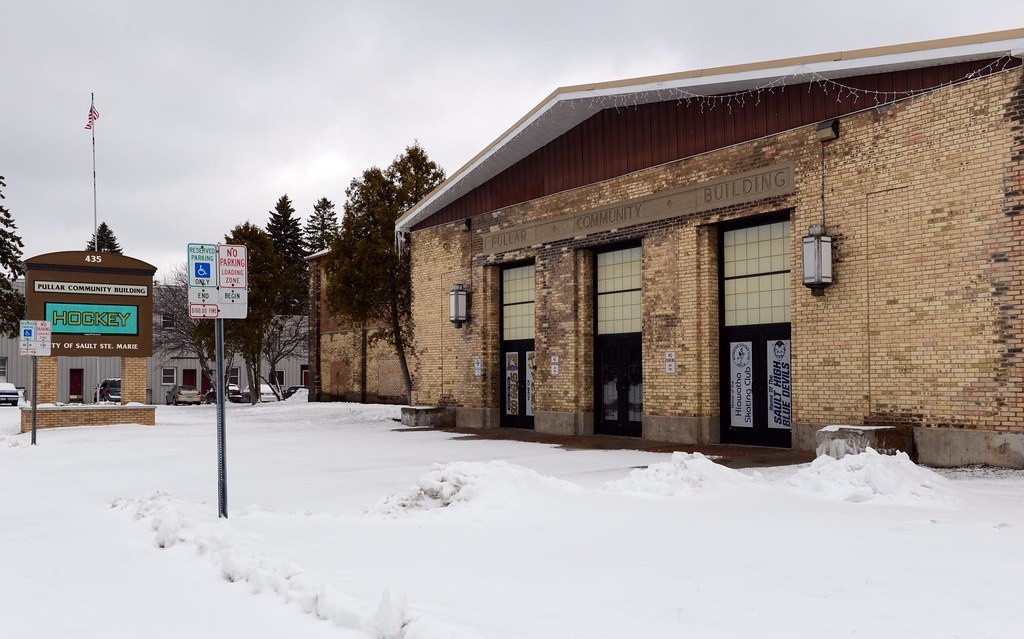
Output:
top-left (205, 383), bottom-right (242, 404)
top-left (0, 383), bottom-right (20, 406)
top-left (240, 383), bottom-right (277, 403)
top-left (93, 377), bottom-right (121, 402)
top-left (284, 385), bottom-right (307, 402)
top-left (166, 385), bottom-right (202, 405)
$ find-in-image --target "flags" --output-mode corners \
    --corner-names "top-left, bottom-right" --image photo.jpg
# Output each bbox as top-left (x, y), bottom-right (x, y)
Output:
top-left (85, 106), bottom-right (100, 129)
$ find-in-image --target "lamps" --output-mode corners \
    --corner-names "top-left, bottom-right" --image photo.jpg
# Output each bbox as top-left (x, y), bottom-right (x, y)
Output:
top-left (449, 284), bottom-right (469, 329)
top-left (802, 224), bottom-right (833, 296)
